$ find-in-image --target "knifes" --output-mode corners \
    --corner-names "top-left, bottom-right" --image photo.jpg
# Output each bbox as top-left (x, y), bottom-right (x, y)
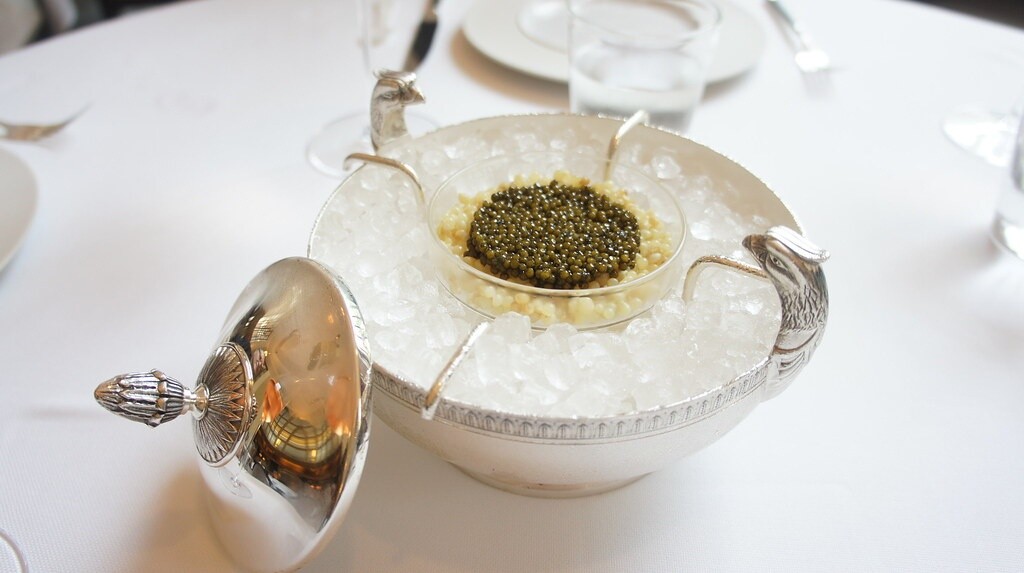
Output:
top-left (397, 0), bottom-right (443, 73)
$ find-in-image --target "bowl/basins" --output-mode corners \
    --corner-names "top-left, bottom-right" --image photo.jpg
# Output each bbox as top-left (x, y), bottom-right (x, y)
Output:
top-left (309, 69), bottom-right (830, 498)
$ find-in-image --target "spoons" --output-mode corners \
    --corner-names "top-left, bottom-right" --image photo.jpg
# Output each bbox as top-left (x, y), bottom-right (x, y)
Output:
top-left (765, 0), bottom-right (832, 74)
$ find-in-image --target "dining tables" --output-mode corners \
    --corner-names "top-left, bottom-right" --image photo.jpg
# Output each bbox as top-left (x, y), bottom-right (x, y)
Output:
top-left (0, 0), bottom-right (1024, 573)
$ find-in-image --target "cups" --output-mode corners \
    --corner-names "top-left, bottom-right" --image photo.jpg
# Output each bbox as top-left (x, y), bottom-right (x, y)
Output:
top-left (567, 0), bottom-right (722, 138)
top-left (984, 106), bottom-right (1024, 266)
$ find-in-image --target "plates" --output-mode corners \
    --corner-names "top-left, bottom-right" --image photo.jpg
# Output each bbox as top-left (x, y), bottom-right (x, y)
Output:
top-left (0, 147), bottom-right (39, 271)
top-left (459, 0), bottom-right (758, 84)
